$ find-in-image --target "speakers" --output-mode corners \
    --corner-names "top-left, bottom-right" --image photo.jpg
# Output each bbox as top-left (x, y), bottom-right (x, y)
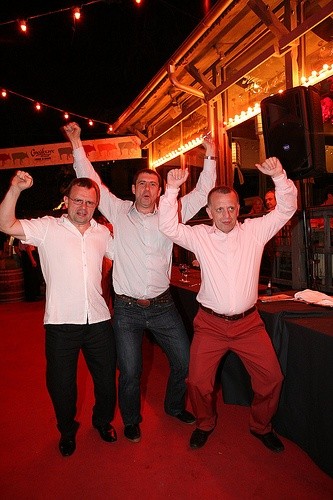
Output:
top-left (260, 86), bottom-right (326, 179)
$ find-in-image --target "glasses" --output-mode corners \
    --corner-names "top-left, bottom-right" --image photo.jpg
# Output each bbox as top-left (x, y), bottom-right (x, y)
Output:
top-left (67, 196), bottom-right (97, 208)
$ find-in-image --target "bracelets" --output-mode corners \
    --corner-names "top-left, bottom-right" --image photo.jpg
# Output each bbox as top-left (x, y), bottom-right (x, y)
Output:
top-left (204, 156), bottom-right (216, 160)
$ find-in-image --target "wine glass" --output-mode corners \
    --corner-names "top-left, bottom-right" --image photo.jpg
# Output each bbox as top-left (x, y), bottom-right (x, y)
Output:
top-left (181, 267), bottom-right (190, 282)
top-left (178, 263), bottom-right (187, 281)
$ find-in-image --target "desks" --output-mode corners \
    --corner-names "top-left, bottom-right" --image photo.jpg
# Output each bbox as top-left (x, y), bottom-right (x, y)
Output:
top-left (220, 290), bottom-right (333, 479)
top-left (144, 264), bottom-right (280, 346)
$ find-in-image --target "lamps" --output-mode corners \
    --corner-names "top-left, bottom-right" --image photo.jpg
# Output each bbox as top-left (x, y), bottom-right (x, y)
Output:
top-left (231, 137), bottom-right (242, 169)
top-left (169, 96), bottom-right (182, 119)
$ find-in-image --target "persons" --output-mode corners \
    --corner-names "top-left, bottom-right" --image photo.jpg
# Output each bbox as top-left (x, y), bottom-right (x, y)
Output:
top-left (265, 189), bottom-right (291, 257)
top-left (249, 197), bottom-right (265, 214)
top-left (63, 122), bottom-right (217, 443)
top-left (8, 236), bottom-right (43, 301)
top-left (159, 157), bottom-right (297, 452)
top-left (0, 171), bottom-right (119, 456)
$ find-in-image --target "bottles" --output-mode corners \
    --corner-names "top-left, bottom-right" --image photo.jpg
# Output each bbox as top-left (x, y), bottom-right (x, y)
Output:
top-left (266, 278), bottom-right (273, 296)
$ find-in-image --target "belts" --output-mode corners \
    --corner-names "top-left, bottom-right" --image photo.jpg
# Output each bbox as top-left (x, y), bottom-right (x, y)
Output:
top-left (119, 287), bottom-right (170, 307)
top-left (124, 424), bottom-right (141, 442)
top-left (94, 424), bottom-right (117, 443)
top-left (199, 303), bottom-right (256, 320)
top-left (251, 430), bottom-right (284, 453)
top-left (165, 409), bottom-right (196, 425)
top-left (191, 427), bottom-right (213, 449)
top-left (59, 432), bottom-right (77, 457)
